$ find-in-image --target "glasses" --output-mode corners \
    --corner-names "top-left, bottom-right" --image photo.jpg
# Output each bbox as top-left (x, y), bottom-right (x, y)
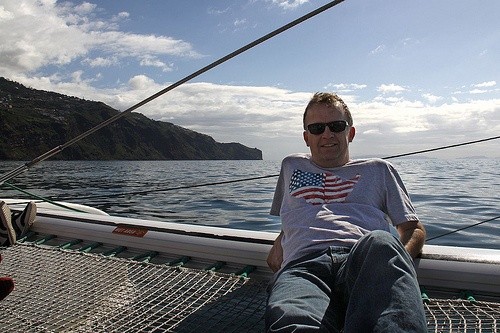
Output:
top-left (304, 120), bottom-right (351, 135)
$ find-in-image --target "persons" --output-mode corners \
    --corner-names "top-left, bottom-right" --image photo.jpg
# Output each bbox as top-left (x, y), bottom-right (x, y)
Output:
top-left (264, 92), bottom-right (427, 333)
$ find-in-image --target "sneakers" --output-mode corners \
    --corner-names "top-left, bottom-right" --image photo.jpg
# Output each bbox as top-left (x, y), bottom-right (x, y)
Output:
top-left (12, 201), bottom-right (37, 240)
top-left (0, 201), bottom-right (17, 248)
